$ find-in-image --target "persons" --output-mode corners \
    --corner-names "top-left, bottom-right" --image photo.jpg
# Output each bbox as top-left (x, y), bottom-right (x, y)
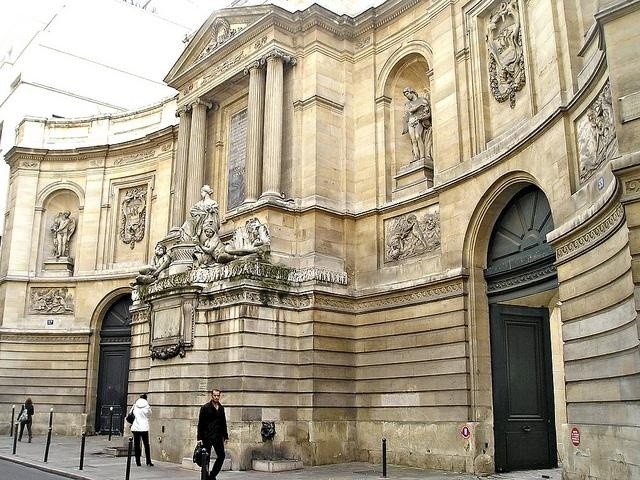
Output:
top-left (401, 86), bottom-right (431, 164)
top-left (16, 397), bottom-right (35, 443)
top-left (196, 388), bottom-right (229, 480)
top-left (50, 209), bottom-right (76, 258)
top-left (191, 224), bottom-right (236, 262)
top-left (134, 242), bottom-right (170, 284)
top-left (387, 214), bottom-right (427, 260)
top-left (127, 393), bottom-right (155, 467)
top-left (181, 184), bottom-right (219, 241)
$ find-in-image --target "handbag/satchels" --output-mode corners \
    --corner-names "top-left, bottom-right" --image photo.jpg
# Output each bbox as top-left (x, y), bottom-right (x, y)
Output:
top-left (126, 408), bottom-right (135, 424)
top-left (17, 404), bottom-right (28, 423)
top-left (192, 442), bottom-right (210, 467)
